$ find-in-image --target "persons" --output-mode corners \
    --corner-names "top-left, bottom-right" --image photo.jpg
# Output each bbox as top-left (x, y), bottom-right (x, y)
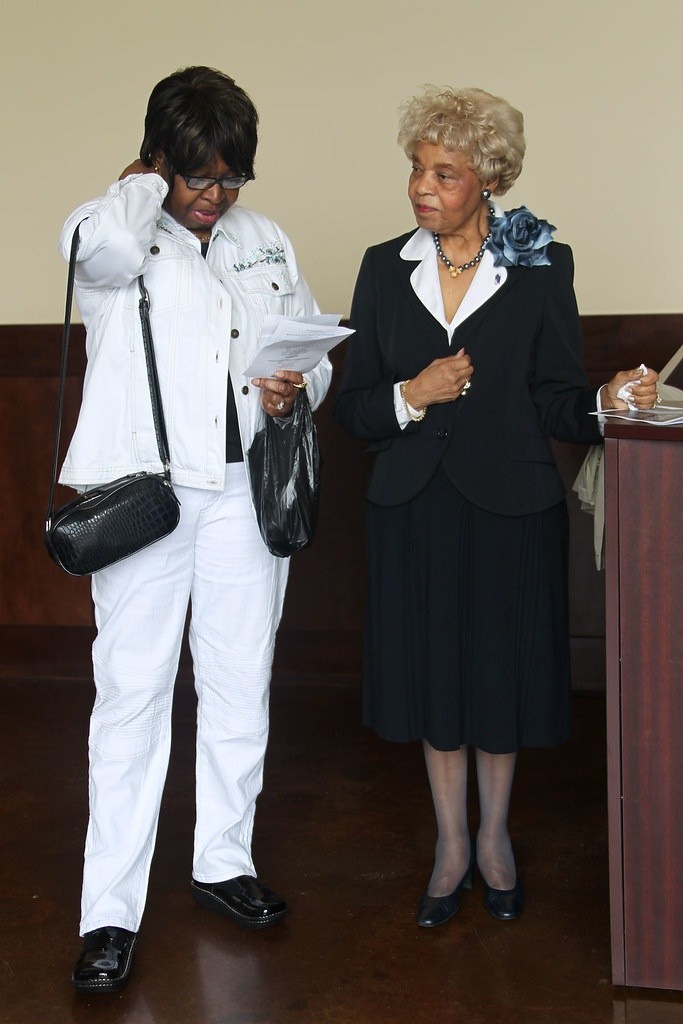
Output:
top-left (60, 66), bottom-right (334, 993)
top-left (336, 83), bottom-right (659, 928)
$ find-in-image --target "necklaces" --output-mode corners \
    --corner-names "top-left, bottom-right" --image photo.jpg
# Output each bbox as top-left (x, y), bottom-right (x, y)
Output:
top-left (433, 208), bottom-right (495, 279)
top-left (194, 232), bottom-right (211, 242)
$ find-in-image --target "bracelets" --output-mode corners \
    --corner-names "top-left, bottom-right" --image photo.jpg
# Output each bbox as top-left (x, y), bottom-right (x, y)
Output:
top-left (402, 379), bottom-right (426, 422)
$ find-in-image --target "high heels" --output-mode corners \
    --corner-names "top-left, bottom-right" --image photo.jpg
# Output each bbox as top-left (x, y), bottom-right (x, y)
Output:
top-left (416, 848), bottom-right (476, 927)
top-left (475, 852), bottom-right (521, 920)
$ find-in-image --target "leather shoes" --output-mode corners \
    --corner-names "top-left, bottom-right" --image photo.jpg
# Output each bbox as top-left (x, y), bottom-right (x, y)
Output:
top-left (71, 909), bottom-right (144, 993)
top-left (191, 879), bottom-right (289, 929)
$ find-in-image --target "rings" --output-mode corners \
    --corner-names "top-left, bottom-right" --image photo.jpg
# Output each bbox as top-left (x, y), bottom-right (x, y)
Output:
top-left (460, 389), bottom-right (467, 396)
top-left (275, 396), bottom-right (285, 411)
top-left (651, 393), bottom-right (662, 410)
top-left (462, 377), bottom-right (472, 390)
top-left (292, 377), bottom-right (308, 389)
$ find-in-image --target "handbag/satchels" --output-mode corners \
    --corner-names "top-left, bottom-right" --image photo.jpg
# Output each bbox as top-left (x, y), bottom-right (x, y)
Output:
top-left (45, 471), bottom-right (182, 577)
top-left (248, 388), bottom-right (320, 557)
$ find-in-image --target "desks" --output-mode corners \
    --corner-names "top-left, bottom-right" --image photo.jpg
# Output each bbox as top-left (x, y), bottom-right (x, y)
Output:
top-left (605, 401), bottom-right (683, 991)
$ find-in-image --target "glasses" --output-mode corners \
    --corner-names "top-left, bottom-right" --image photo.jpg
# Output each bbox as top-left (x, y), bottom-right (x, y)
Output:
top-left (178, 173), bottom-right (252, 191)
